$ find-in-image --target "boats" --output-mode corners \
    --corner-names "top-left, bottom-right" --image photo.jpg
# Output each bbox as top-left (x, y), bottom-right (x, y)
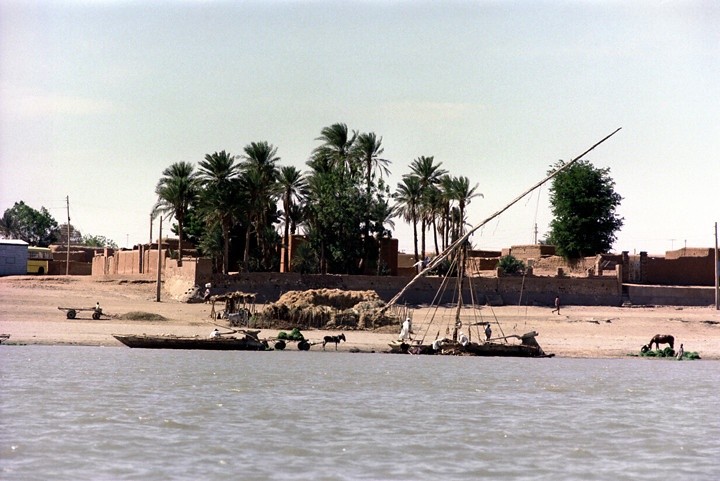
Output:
top-left (112, 328), bottom-right (272, 351)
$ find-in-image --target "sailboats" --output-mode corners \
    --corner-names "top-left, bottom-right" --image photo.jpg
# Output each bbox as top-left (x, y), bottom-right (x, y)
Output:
top-left (385, 200), bottom-right (556, 359)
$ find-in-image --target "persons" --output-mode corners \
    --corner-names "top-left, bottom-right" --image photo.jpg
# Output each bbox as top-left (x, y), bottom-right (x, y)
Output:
top-left (459, 332), bottom-right (468, 346)
top-left (641, 344), bottom-right (650, 353)
top-left (675, 343), bottom-right (683, 359)
top-left (399, 318), bottom-right (413, 341)
top-left (433, 339), bottom-right (442, 352)
top-left (485, 324), bottom-right (492, 341)
top-left (204, 287), bottom-right (211, 304)
top-left (552, 296), bottom-right (561, 315)
top-left (95, 302), bottom-right (105, 315)
top-left (210, 328), bottom-right (220, 338)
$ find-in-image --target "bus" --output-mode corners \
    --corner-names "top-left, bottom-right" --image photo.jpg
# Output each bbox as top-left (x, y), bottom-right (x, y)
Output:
top-left (27, 246), bottom-right (49, 275)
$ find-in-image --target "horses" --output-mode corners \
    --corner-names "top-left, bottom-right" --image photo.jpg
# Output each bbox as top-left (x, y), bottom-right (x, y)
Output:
top-left (323, 333), bottom-right (347, 352)
top-left (641, 334), bottom-right (676, 353)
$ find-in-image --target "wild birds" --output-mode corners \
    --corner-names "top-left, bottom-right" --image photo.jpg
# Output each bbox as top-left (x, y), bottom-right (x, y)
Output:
top-left (484, 323), bottom-right (492, 341)
